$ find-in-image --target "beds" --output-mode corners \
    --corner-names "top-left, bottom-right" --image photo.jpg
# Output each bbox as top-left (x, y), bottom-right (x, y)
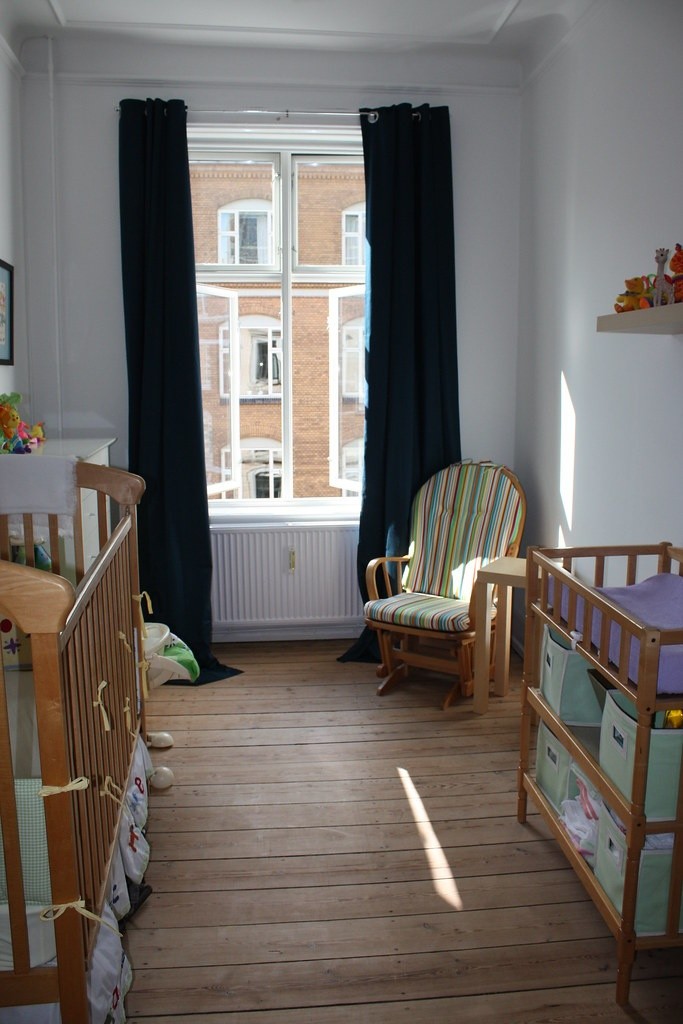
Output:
top-left (0, 455), bottom-right (158, 1024)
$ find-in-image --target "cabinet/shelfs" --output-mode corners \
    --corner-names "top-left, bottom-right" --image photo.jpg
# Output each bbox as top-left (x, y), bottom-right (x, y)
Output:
top-left (506, 541), bottom-right (683, 1005)
top-left (0, 437), bottom-right (118, 568)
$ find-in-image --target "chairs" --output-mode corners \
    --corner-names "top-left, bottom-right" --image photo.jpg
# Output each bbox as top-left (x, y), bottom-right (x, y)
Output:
top-left (364, 463), bottom-right (525, 712)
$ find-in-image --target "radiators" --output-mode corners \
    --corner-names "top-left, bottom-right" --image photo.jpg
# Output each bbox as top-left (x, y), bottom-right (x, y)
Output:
top-left (210, 520), bottom-right (364, 628)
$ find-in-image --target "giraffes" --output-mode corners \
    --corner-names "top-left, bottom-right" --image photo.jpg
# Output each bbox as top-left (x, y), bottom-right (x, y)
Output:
top-left (653, 247), bottom-right (675, 307)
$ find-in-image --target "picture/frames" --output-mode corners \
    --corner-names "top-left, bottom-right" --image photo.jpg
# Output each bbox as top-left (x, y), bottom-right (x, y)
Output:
top-left (0, 259), bottom-right (15, 366)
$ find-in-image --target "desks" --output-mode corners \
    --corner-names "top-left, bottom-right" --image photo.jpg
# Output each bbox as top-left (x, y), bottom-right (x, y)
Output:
top-left (473, 556), bottom-right (562, 715)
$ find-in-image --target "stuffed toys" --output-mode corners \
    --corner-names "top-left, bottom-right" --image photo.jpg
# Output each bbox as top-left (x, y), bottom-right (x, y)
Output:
top-left (614, 243), bottom-right (683, 312)
top-left (0, 392), bottom-right (46, 455)
